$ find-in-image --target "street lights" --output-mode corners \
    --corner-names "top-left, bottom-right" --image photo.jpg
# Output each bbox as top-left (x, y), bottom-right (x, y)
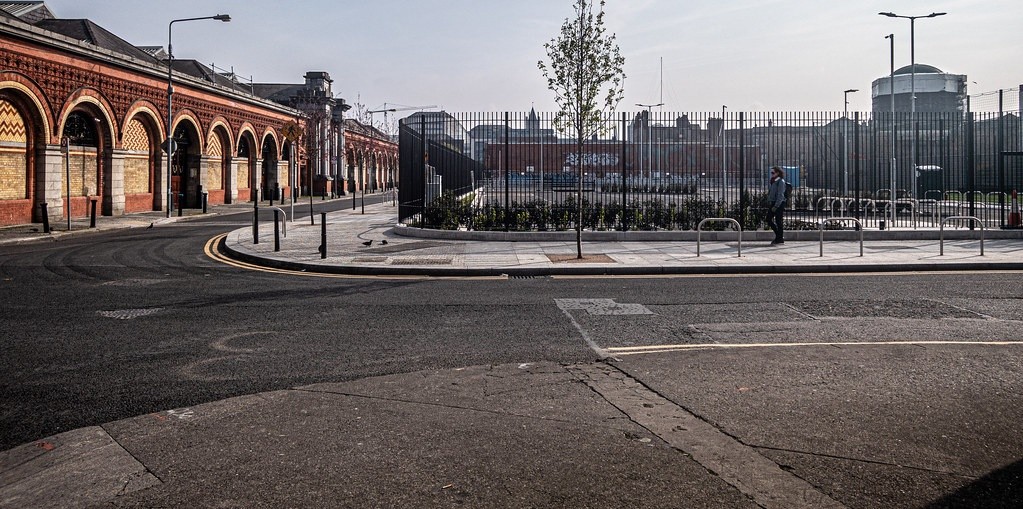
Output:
top-left (166, 13), bottom-right (233, 218)
top-left (722, 105), bottom-right (727, 211)
top-left (843, 89), bottom-right (860, 209)
top-left (636, 103), bottom-right (664, 191)
top-left (878, 12), bottom-right (947, 214)
top-left (885, 33), bottom-right (897, 226)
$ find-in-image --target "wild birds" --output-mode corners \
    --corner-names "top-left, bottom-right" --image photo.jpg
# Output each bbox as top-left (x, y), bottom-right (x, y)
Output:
top-left (362, 240), bottom-right (373, 247)
top-left (382, 239), bottom-right (388, 244)
top-left (146, 222), bottom-right (154, 230)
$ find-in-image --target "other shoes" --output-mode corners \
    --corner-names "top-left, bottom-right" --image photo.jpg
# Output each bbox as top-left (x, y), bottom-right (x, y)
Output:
top-left (771, 237), bottom-right (784, 244)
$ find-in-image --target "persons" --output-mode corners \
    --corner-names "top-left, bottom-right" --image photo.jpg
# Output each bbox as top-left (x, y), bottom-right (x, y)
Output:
top-left (766, 167), bottom-right (786, 245)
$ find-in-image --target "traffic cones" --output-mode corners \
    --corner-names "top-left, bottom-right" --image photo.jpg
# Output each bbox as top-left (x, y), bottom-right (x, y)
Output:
top-left (1000, 189), bottom-right (1023, 229)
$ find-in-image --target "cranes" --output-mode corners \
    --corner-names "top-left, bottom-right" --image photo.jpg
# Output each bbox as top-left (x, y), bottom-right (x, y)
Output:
top-left (366, 102), bottom-right (438, 132)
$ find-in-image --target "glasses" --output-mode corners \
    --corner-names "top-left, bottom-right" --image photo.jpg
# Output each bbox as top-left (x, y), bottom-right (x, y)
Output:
top-left (770, 172), bottom-right (775, 174)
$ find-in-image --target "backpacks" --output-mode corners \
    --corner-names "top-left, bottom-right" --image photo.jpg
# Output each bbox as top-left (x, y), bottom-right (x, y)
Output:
top-left (777, 179), bottom-right (792, 199)
